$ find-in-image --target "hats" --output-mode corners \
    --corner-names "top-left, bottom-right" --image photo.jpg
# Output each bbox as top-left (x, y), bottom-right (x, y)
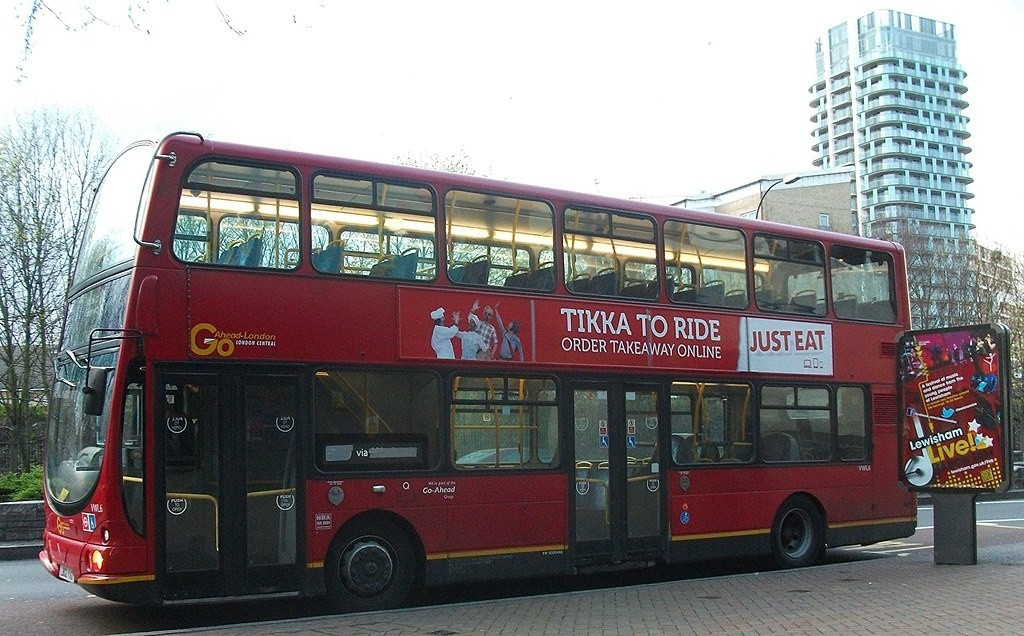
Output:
top-left (430, 307), bottom-right (446, 321)
top-left (468, 314), bottom-right (480, 332)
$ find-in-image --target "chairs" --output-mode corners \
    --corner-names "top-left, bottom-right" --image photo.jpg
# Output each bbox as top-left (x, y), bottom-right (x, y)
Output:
top-left (643, 273), bottom-right (675, 300)
top-left (697, 280), bottom-right (725, 306)
top-left (312, 247), bottom-right (322, 271)
top-left (503, 267), bottom-right (531, 288)
top-left (387, 247), bottom-right (420, 279)
top-left (588, 267), bottom-right (616, 295)
top-left (755, 285), bottom-right (774, 309)
top-left (320, 240), bottom-right (344, 273)
top-left (370, 254), bottom-right (399, 277)
top-left (567, 272), bottom-right (592, 293)
top-left (230, 232), bottom-right (263, 267)
top-left (623, 279), bottom-right (647, 298)
top-left (217, 240), bottom-right (243, 264)
top-left (448, 261), bottom-right (469, 282)
top-left (775, 289), bottom-right (858, 318)
top-left (462, 255), bottom-right (491, 285)
top-left (575, 432), bottom-right (863, 516)
top-left (674, 284), bottom-right (698, 302)
top-left (527, 261), bottom-right (554, 289)
top-left (724, 288), bottom-right (745, 307)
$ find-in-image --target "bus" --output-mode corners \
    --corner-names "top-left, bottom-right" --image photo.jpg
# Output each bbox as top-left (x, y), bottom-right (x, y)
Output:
top-left (36, 133), bottom-right (922, 613)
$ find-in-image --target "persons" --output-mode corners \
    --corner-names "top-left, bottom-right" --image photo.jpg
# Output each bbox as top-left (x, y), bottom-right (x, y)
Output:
top-left (429, 298), bottom-right (525, 362)
top-left (671, 434), bottom-right (721, 464)
top-left (793, 419), bottom-right (815, 447)
